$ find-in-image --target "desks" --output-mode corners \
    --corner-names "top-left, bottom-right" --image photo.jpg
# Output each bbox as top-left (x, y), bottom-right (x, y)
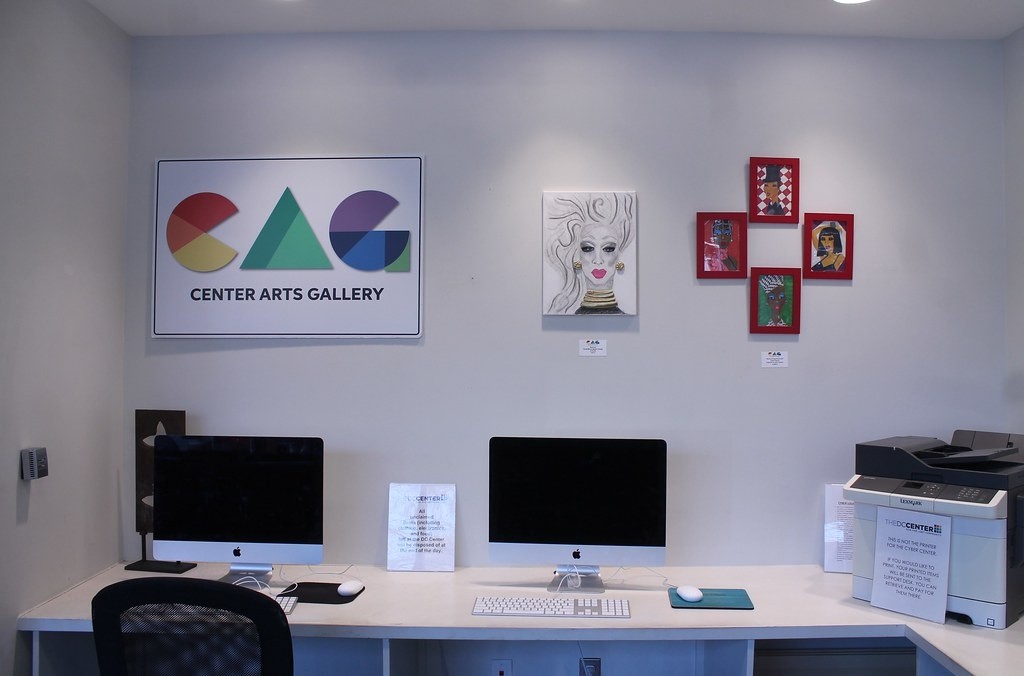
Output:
top-left (16, 562), bottom-right (1024, 676)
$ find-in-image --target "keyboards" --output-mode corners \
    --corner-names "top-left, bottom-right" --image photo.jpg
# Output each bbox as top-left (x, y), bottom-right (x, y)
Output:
top-left (268, 596), bottom-right (299, 615)
top-left (471, 595), bottom-right (632, 619)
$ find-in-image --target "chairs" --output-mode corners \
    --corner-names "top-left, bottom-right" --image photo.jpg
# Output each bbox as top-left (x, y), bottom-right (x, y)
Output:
top-left (90, 576), bottom-right (295, 676)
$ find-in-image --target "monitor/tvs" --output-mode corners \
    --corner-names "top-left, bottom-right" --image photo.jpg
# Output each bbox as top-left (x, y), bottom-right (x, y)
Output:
top-left (153, 434), bottom-right (327, 588)
top-left (488, 435), bottom-right (669, 593)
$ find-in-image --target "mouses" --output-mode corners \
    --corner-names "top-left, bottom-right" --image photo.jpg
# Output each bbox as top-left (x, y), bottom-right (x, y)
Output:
top-left (336, 580), bottom-right (364, 597)
top-left (676, 584), bottom-right (704, 603)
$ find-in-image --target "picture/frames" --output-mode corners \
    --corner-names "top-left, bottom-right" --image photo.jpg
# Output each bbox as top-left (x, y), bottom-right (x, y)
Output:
top-left (748, 157), bottom-right (800, 224)
top-left (802, 212), bottom-right (855, 280)
top-left (749, 267), bottom-right (801, 334)
top-left (697, 212), bottom-right (748, 279)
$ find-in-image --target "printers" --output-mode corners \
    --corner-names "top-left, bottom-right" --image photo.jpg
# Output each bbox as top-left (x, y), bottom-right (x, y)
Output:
top-left (843, 426), bottom-right (1024, 630)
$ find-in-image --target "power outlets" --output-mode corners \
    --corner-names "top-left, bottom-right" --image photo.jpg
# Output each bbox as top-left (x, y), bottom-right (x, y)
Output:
top-left (578, 658), bottom-right (601, 676)
top-left (492, 659), bottom-right (513, 676)
